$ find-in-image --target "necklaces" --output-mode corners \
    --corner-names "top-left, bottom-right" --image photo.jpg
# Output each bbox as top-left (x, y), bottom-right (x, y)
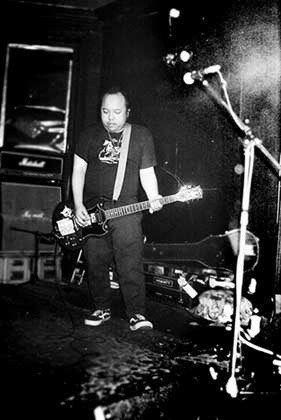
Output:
top-left (105, 129), bottom-right (125, 155)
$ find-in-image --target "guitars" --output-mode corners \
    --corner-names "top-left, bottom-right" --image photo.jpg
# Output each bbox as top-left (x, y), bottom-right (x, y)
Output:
top-left (51, 184), bottom-right (204, 251)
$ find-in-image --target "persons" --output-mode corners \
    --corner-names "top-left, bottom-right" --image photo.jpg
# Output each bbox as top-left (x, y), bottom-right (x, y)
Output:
top-left (71, 85), bottom-right (164, 332)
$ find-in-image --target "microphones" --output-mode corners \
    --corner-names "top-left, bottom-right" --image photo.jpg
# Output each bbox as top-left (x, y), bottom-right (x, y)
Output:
top-left (183, 65), bottom-right (221, 84)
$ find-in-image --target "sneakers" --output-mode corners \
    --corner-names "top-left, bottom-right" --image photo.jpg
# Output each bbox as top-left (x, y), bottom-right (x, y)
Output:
top-left (128, 310), bottom-right (154, 333)
top-left (84, 306), bottom-right (112, 327)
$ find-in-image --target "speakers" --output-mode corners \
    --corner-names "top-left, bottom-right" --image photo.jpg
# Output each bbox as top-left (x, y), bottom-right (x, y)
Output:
top-left (0, 182), bottom-right (62, 255)
